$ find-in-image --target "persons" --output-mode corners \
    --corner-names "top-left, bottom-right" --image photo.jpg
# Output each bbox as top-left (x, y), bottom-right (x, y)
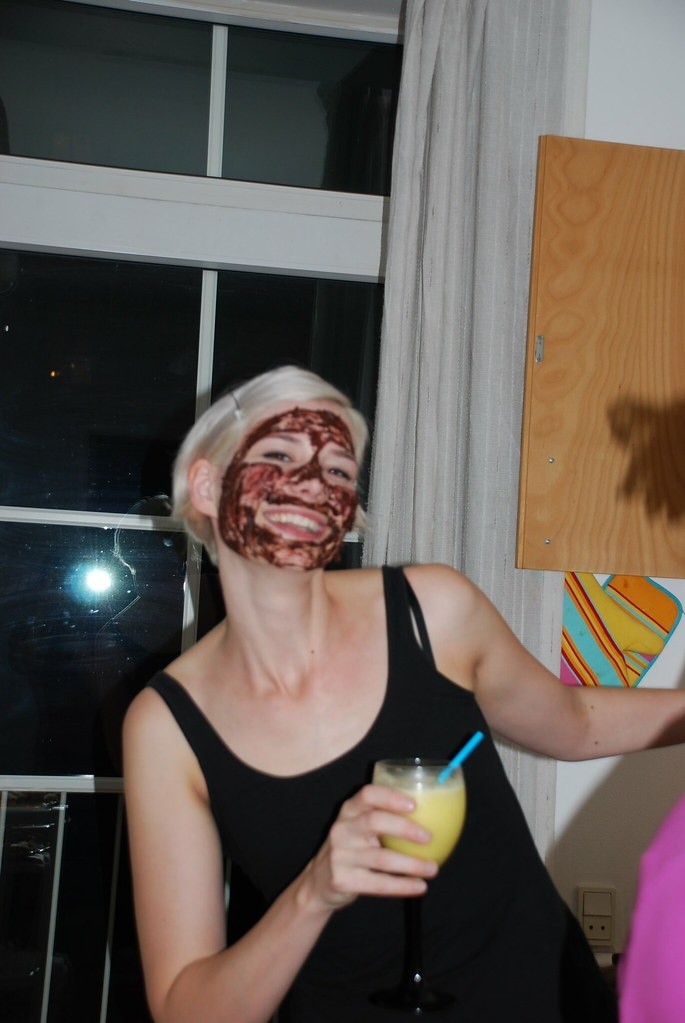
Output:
top-left (121, 363), bottom-right (685, 1023)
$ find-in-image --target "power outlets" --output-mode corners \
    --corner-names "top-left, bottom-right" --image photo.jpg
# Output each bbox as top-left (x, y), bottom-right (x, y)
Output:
top-left (574, 886), bottom-right (616, 955)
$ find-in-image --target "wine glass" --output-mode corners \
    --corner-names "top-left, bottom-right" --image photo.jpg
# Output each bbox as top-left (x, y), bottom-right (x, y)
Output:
top-left (373, 760), bottom-right (465, 1015)
